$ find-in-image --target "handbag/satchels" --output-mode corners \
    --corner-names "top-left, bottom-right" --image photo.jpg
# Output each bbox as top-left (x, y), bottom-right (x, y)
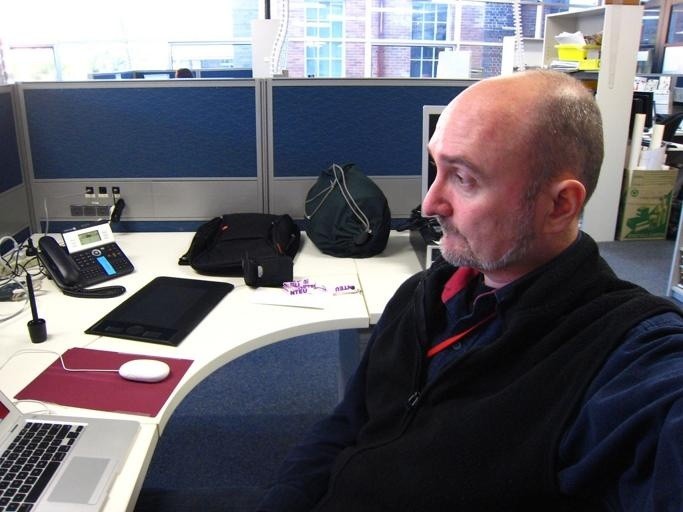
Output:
top-left (178, 213), bottom-right (302, 277)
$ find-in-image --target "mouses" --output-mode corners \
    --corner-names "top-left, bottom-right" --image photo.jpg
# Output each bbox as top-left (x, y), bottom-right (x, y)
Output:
top-left (117, 356), bottom-right (172, 383)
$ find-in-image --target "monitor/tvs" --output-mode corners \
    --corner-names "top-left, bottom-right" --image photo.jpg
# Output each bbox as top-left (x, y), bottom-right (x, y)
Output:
top-left (628, 91), bottom-right (654, 140)
top-left (140, 71), bottom-right (170, 82)
top-left (420, 104), bottom-right (447, 219)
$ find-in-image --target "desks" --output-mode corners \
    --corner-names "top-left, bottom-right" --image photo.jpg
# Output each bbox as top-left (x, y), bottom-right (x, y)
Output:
top-left (0, 222), bottom-right (425, 512)
top-left (636, 125), bottom-right (683, 164)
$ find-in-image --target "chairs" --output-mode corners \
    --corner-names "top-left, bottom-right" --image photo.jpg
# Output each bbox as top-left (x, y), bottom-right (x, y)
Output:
top-left (655, 111), bottom-right (682, 141)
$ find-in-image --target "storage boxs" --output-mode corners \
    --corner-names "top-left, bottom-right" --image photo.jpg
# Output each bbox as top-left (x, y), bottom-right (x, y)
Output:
top-left (620, 146), bottom-right (679, 243)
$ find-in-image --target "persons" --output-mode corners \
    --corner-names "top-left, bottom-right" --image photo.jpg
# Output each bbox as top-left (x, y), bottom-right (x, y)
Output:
top-left (253, 69), bottom-right (683, 512)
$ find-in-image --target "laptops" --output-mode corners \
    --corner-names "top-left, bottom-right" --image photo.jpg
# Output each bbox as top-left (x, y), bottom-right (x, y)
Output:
top-left (0, 390), bottom-right (142, 512)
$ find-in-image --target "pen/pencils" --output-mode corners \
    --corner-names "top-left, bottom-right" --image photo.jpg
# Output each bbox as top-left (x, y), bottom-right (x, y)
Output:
top-left (27, 273), bottom-right (38, 322)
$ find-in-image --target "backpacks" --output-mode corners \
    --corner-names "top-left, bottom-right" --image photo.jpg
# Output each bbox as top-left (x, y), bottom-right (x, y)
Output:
top-left (305, 161), bottom-right (392, 257)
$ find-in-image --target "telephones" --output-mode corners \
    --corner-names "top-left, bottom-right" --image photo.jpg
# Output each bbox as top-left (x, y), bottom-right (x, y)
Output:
top-left (37, 220), bottom-right (134, 290)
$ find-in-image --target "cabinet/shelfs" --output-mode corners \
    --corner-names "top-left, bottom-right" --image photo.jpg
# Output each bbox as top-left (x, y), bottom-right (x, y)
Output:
top-left (541, 3), bottom-right (646, 243)
